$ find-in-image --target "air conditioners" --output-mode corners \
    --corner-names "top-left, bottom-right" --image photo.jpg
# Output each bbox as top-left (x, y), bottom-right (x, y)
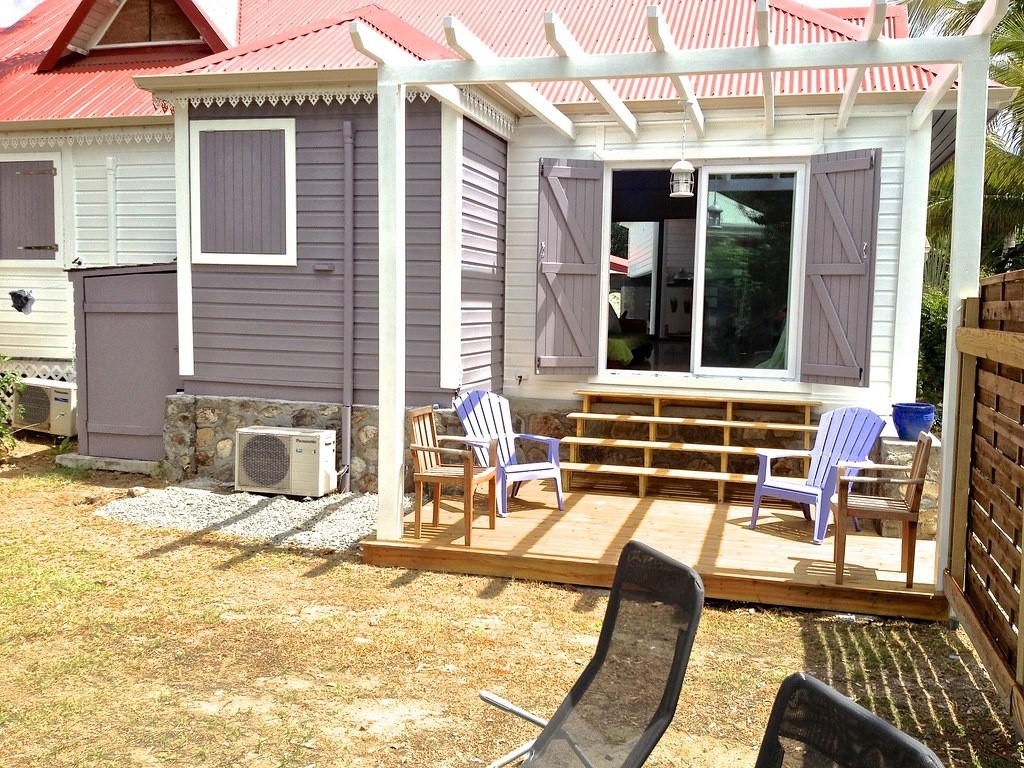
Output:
top-left (235, 425), bottom-right (338, 498)
top-left (12, 377), bottom-right (78, 437)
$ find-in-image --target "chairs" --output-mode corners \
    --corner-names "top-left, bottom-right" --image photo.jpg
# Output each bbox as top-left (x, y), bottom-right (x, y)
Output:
top-left (755, 672), bottom-right (944, 768)
top-left (828, 430), bottom-right (932, 589)
top-left (452, 389), bottom-right (565, 518)
top-left (748, 405), bottom-right (886, 545)
top-left (478, 538), bottom-right (705, 768)
top-left (407, 405), bottom-right (500, 547)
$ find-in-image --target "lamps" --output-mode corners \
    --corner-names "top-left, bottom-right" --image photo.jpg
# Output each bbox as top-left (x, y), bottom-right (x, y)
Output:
top-left (707, 175), bottom-right (723, 229)
top-left (669, 101), bottom-right (695, 197)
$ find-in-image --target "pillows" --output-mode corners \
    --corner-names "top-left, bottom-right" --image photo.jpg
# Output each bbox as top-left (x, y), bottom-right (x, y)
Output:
top-left (609, 301), bottom-right (622, 333)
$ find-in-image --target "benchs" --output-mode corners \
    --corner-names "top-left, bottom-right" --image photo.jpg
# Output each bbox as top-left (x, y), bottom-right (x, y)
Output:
top-left (560, 390), bottom-right (822, 505)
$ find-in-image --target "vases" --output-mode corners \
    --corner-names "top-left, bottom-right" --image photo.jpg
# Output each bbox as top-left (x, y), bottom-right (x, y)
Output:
top-left (892, 403), bottom-right (935, 442)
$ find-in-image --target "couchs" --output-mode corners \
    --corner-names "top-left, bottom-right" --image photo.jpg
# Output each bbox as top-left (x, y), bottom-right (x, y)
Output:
top-left (607, 318), bottom-right (649, 370)
